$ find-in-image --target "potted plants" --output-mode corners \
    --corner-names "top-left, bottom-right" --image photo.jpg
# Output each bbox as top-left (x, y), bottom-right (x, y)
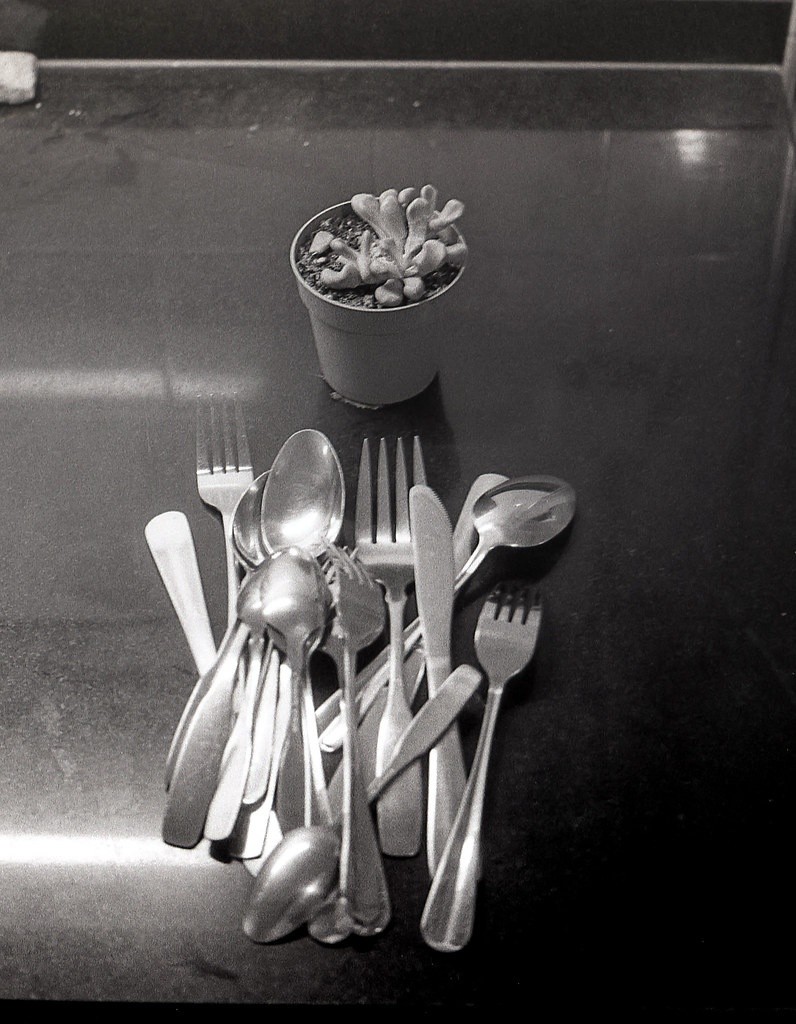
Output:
top-left (287, 183), bottom-right (471, 405)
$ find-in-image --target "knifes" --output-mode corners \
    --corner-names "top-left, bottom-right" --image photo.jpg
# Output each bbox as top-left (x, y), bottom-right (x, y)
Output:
top-left (408, 483), bottom-right (466, 877)
top-left (145, 512), bottom-right (283, 874)
top-left (326, 473), bottom-right (535, 814)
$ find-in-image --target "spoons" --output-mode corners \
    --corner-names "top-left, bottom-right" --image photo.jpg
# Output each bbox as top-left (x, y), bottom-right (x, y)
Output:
top-left (161, 469), bottom-right (269, 848)
top-left (203, 577), bottom-right (264, 841)
top-left (244, 429), bottom-right (344, 805)
top-left (265, 620), bottom-right (331, 826)
top-left (319, 476), bottom-right (576, 750)
top-left (259, 546), bottom-right (325, 837)
top-left (242, 666), bottom-right (483, 943)
top-left (164, 543), bottom-right (256, 788)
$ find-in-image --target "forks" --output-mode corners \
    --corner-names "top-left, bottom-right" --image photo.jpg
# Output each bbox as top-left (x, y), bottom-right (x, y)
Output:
top-left (420, 582), bottom-right (543, 950)
top-left (356, 436), bottom-right (426, 856)
top-left (318, 540), bottom-right (389, 934)
top-left (320, 546), bottom-right (359, 608)
top-left (195, 392), bottom-right (255, 627)
